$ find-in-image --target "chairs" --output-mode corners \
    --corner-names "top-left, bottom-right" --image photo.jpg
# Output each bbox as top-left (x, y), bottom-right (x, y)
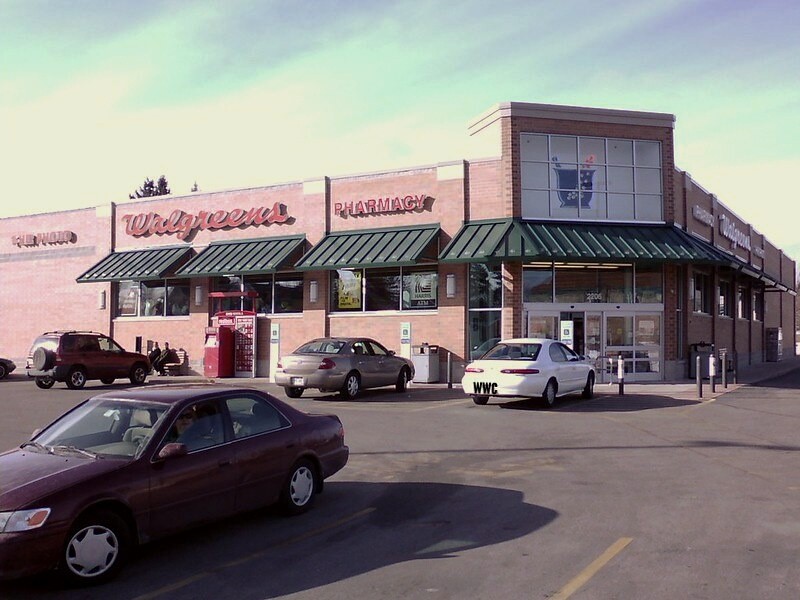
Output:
top-left (354, 345), bottom-right (364, 354)
top-left (325, 344), bottom-right (336, 352)
top-left (129, 408), bottom-right (158, 428)
top-left (236, 402), bottom-right (282, 438)
top-left (195, 399), bottom-right (224, 444)
top-left (510, 347), bottom-right (523, 358)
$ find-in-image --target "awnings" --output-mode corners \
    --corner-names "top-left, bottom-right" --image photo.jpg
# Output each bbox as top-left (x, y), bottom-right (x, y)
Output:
top-left (438, 219), bottom-right (727, 263)
top-left (679, 230), bottom-right (798, 296)
top-left (75, 246), bottom-right (192, 283)
top-left (174, 233), bottom-right (306, 278)
top-left (293, 223), bottom-right (441, 269)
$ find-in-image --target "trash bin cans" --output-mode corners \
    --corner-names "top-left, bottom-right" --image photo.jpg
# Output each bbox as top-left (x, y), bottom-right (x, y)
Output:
top-left (690, 341), bottom-right (715, 379)
top-left (412, 342), bottom-right (440, 383)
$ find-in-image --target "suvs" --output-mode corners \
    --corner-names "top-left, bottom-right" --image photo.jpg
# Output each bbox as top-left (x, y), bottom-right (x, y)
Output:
top-left (23, 330), bottom-right (153, 389)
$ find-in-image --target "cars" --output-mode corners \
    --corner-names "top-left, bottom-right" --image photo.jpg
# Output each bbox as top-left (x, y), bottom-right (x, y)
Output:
top-left (274, 337), bottom-right (416, 398)
top-left (583, 339), bottom-right (619, 370)
top-left (0, 359), bottom-right (16, 379)
top-left (471, 337), bottom-right (502, 360)
top-left (0, 379), bottom-right (349, 590)
top-left (461, 337), bottom-right (598, 403)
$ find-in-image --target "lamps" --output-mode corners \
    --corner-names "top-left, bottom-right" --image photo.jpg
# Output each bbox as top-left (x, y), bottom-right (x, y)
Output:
top-left (717, 287), bottom-right (720, 304)
top-left (310, 280), bottom-right (318, 303)
top-left (446, 275), bottom-right (456, 298)
top-left (195, 286), bottom-right (202, 306)
top-left (688, 279), bottom-right (694, 300)
top-left (99, 290), bottom-right (105, 308)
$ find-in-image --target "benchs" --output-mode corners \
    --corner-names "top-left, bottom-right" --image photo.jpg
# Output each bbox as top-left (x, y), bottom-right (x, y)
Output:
top-left (148, 351), bottom-right (186, 376)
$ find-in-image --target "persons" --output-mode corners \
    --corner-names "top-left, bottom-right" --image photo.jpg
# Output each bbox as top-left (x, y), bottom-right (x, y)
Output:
top-left (165, 407), bottom-right (208, 451)
top-left (146, 341), bottom-right (173, 377)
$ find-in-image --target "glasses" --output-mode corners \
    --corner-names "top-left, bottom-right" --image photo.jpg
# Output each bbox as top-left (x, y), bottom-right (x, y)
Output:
top-left (177, 412), bottom-right (192, 420)
